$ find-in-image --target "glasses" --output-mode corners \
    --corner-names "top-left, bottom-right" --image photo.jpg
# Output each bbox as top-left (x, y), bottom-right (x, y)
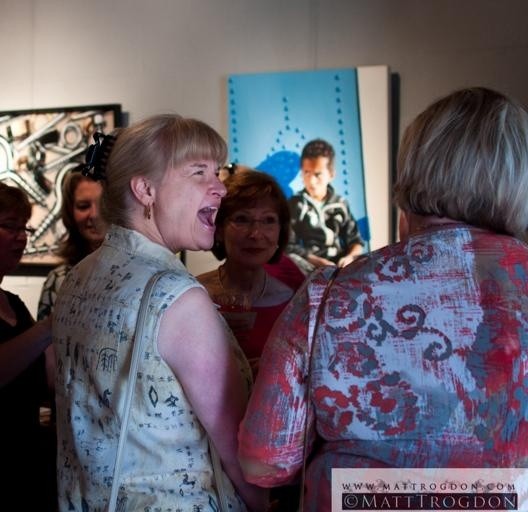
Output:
top-left (1, 224), bottom-right (35, 236)
top-left (229, 213), bottom-right (280, 231)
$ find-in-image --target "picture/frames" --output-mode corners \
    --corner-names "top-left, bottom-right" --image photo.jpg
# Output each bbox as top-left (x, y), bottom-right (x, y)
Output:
top-left (0, 103), bottom-right (122, 277)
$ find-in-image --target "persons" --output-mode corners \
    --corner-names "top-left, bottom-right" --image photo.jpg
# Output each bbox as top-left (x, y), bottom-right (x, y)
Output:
top-left (0, 187), bottom-right (59, 511)
top-left (238, 86), bottom-right (527, 511)
top-left (209, 163), bottom-right (306, 291)
top-left (187, 169), bottom-right (309, 374)
top-left (284, 140), bottom-right (366, 276)
top-left (35, 166), bottom-right (113, 384)
top-left (42, 112), bottom-right (276, 509)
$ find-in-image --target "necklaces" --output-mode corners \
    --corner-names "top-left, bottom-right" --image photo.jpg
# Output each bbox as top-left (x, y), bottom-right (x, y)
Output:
top-left (216, 261), bottom-right (269, 308)
